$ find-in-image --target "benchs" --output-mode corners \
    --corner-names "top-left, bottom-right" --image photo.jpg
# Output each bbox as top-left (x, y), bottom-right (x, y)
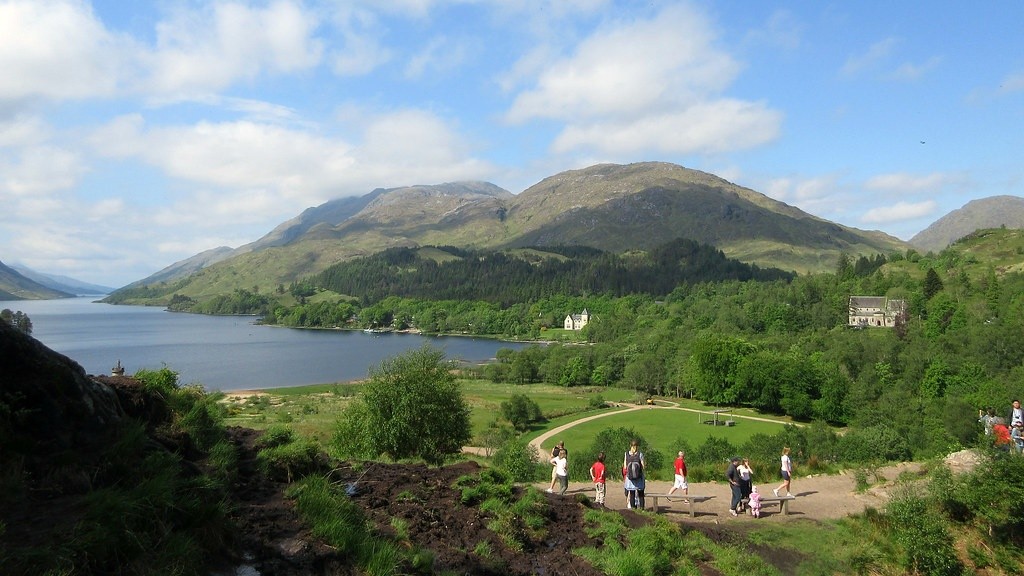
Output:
top-left (643, 493), bottom-right (711, 518)
top-left (725, 421), bottom-right (735, 427)
top-left (741, 495), bottom-right (795, 517)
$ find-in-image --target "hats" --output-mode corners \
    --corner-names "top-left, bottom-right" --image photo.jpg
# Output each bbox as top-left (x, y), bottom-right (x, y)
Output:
top-left (732, 457), bottom-right (740, 462)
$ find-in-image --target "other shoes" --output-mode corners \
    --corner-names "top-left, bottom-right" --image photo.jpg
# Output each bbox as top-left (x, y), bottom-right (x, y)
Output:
top-left (773, 489), bottom-right (779, 497)
top-left (665, 497), bottom-right (671, 502)
top-left (545, 489), bottom-right (554, 493)
top-left (729, 509), bottom-right (738, 517)
top-left (684, 501), bottom-right (691, 505)
top-left (787, 494), bottom-right (794, 498)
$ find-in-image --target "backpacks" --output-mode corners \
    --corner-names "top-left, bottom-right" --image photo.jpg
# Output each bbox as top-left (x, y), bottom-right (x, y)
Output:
top-left (627, 451), bottom-right (641, 480)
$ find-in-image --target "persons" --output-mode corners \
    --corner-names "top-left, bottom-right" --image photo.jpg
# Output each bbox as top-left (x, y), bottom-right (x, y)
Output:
top-left (725, 457), bottom-right (753, 517)
top-left (550, 449), bottom-right (568, 496)
top-left (545, 440), bottom-right (568, 493)
top-left (978, 399), bottom-right (1024, 454)
top-left (589, 452), bottom-right (606, 507)
top-left (666, 451), bottom-right (690, 504)
top-left (772, 446), bottom-right (795, 498)
top-left (749, 485), bottom-right (764, 519)
top-left (622, 439), bottom-right (646, 511)
